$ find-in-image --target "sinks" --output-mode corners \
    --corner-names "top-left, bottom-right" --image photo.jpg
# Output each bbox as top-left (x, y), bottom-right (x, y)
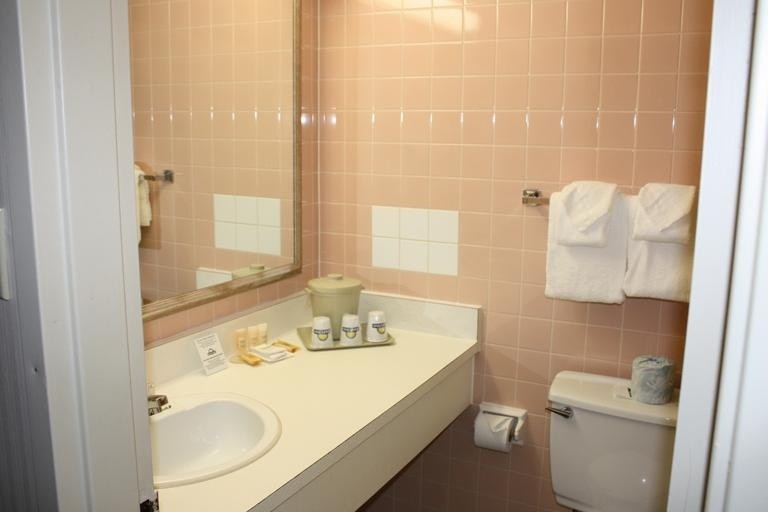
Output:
top-left (146, 392), bottom-right (282, 489)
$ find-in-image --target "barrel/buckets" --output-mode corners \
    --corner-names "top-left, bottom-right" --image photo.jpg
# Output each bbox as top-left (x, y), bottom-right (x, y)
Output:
top-left (304, 271), bottom-right (364, 341)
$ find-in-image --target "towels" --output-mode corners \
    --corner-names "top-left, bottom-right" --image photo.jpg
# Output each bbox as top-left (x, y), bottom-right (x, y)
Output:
top-left (543, 180), bottom-right (696, 306)
top-left (133, 164), bottom-right (153, 245)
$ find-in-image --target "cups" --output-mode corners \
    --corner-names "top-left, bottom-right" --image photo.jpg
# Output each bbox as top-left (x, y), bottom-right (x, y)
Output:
top-left (310, 310), bottom-right (389, 349)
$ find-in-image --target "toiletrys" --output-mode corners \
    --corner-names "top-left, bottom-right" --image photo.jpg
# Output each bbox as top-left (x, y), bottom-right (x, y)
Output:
top-left (234, 322), bottom-right (268, 351)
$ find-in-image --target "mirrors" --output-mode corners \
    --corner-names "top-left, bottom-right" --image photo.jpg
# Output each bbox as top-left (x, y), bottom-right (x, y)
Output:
top-left (127, 0), bottom-right (304, 321)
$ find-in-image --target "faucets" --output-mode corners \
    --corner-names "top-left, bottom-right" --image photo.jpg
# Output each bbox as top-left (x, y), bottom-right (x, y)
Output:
top-left (146, 394), bottom-right (171, 417)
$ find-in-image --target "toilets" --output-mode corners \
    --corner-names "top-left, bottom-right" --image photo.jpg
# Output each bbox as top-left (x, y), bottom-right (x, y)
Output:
top-left (545, 370), bottom-right (679, 512)
